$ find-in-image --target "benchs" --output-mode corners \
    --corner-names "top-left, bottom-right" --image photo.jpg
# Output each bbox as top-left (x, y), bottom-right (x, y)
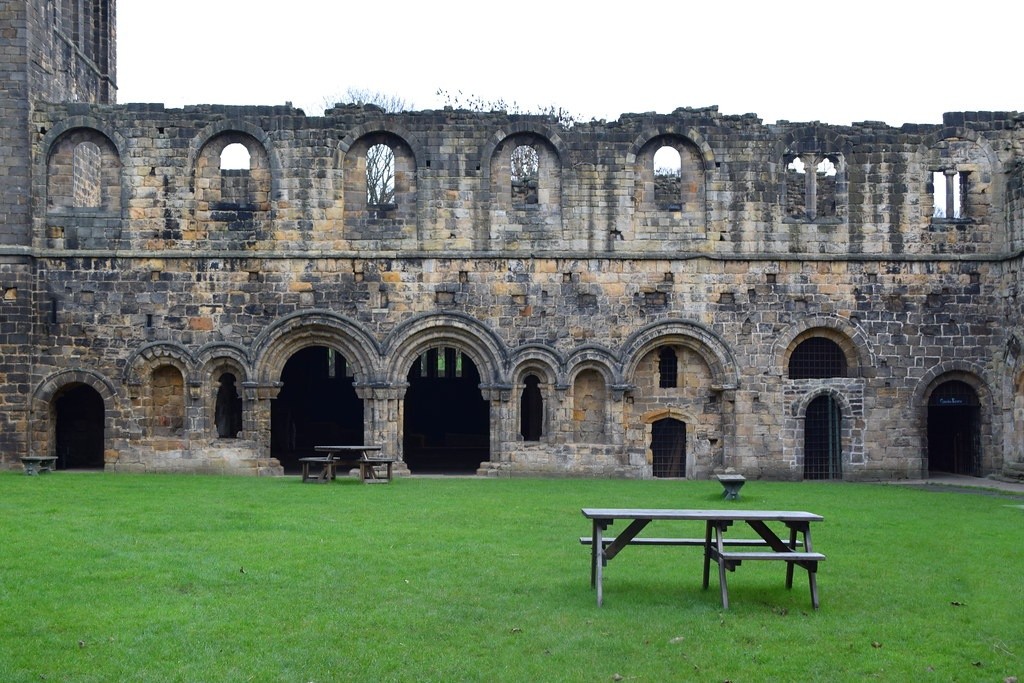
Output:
top-left (720, 552), bottom-right (826, 611)
top-left (717, 474), bottom-right (747, 500)
top-left (579, 537), bottom-right (804, 607)
top-left (357, 458), bottom-right (395, 483)
top-left (299, 457), bottom-right (340, 483)
top-left (19, 456), bottom-right (58, 476)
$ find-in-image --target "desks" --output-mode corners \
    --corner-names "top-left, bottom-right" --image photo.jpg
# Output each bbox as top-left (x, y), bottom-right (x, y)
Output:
top-left (314, 446), bottom-right (382, 483)
top-left (582, 508), bottom-right (824, 553)
